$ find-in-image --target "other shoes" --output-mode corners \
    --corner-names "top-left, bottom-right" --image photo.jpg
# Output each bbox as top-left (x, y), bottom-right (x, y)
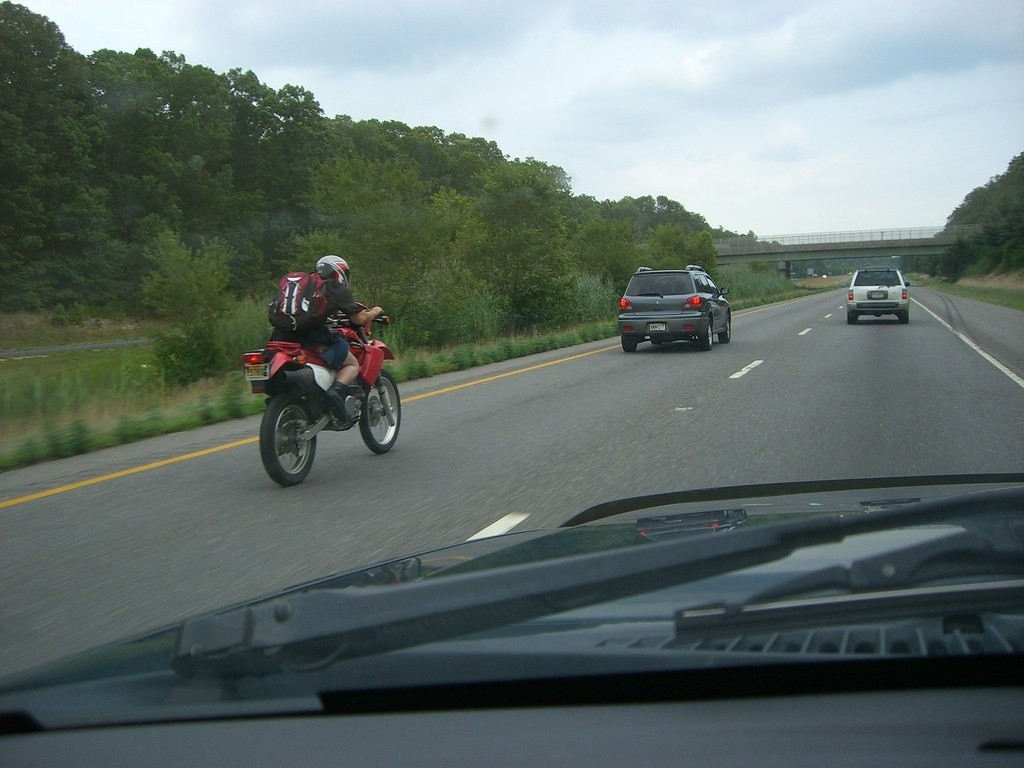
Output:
top-left (324, 389), bottom-right (346, 419)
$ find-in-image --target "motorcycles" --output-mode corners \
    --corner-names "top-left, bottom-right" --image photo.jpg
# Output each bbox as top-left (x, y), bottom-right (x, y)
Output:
top-left (241, 302), bottom-right (400, 486)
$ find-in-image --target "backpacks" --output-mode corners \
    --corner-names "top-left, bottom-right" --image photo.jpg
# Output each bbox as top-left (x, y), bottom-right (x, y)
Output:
top-left (269, 271), bottom-right (329, 332)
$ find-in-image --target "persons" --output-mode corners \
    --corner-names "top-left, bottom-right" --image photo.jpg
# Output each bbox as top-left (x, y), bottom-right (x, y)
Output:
top-left (270, 255), bottom-right (384, 421)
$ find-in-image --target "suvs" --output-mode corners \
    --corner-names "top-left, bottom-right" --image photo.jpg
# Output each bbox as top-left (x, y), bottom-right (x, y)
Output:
top-left (618, 265), bottom-right (731, 352)
top-left (847, 269), bottom-right (910, 324)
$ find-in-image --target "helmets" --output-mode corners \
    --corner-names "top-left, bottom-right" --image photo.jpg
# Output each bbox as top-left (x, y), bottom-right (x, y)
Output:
top-left (316, 254), bottom-right (351, 288)
